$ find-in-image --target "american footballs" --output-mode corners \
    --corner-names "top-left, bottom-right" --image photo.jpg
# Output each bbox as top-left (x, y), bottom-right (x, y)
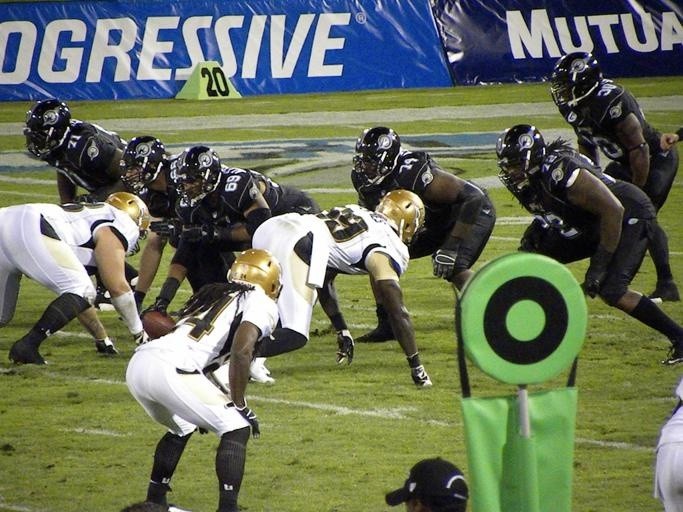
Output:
top-left (140, 309), bottom-right (175, 338)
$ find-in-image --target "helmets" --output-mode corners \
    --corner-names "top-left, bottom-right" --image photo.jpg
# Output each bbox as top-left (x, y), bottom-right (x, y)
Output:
top-left (225, 248), bottom-right (284, 301)
top-left (108, 191), bottom-right (150, 239)
top-left (117, 134), bottom-right (169, 191)
top-left (493, 123), bottom-right (545, 195)
top-left (21, 98), bottom-right (71, 161)
top-left (549, 51), bottom-right (606, 109)
top-left (351, 125), bottom-right (402, 186)
top-left (376, 189), bottom-right (429, 245)
top-left (167, 144), bottom-right (221, 207)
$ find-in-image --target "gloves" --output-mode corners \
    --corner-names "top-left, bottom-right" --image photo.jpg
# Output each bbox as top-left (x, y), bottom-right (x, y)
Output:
top-left (235, 399), bottom-right (261, 441)
top-left (149, 220), bottom-right (182, 238)
top-left (135, 330), bottom-right (152, 346)
top-left (95, 336), bottom-right (118, 357)
top-left (181, 222), bottom-right (213, 241)
top-left (586, 276), bottom-right (599, 298)
top-left (430, 249), bottom-right (456, 281)
top-left (139, 294), bottom-right (170, 319)
top-left (334, 329), bottom-right (355, 366)
top-left (409, 364), bottom-right (431, 389)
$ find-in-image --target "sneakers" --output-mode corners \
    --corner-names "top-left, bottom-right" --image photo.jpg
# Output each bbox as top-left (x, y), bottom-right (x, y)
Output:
top-left (8, 338), bottom-right (49, 367)
top-left (212, 361), bottom-right (232, 392)
top-left (663, 332), bottom-right (683, 366)
top-left (91, 271), bottom-right (141, 308)
top-left (249, 357), bottom-right (277, 385)
top-left (643, 273), bottom-right (681, 304)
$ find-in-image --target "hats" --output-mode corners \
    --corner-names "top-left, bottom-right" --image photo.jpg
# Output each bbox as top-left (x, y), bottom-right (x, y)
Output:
top-left (385, 458), bottom-right (469, 506)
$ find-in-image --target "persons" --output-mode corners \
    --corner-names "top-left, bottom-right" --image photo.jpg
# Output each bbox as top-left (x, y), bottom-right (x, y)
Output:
top-left (385, 457), bottom-right (468, 512)
top-left (127, 250), bottom-right (282, 512)
top-left (212, 189), bottom-right (434, 393)
top-left (23, 99), bottom-right (143, 312)
top-left (0, 191), bottom-right (151, 364)
top-left (654, 375), bottom-right (683, 512)
top-left (494, 124), bottom-right (681, 366)
top-left (350, 128), bottom-right (495, 343)
top-left (660, 126), bottom-right (682, 152)
top-left (140, 144), bottom-right (321, 318)
top-left (119, 136), bottom-right (215, 312)
top-left (547, 51), bottom-right (680, 301)
